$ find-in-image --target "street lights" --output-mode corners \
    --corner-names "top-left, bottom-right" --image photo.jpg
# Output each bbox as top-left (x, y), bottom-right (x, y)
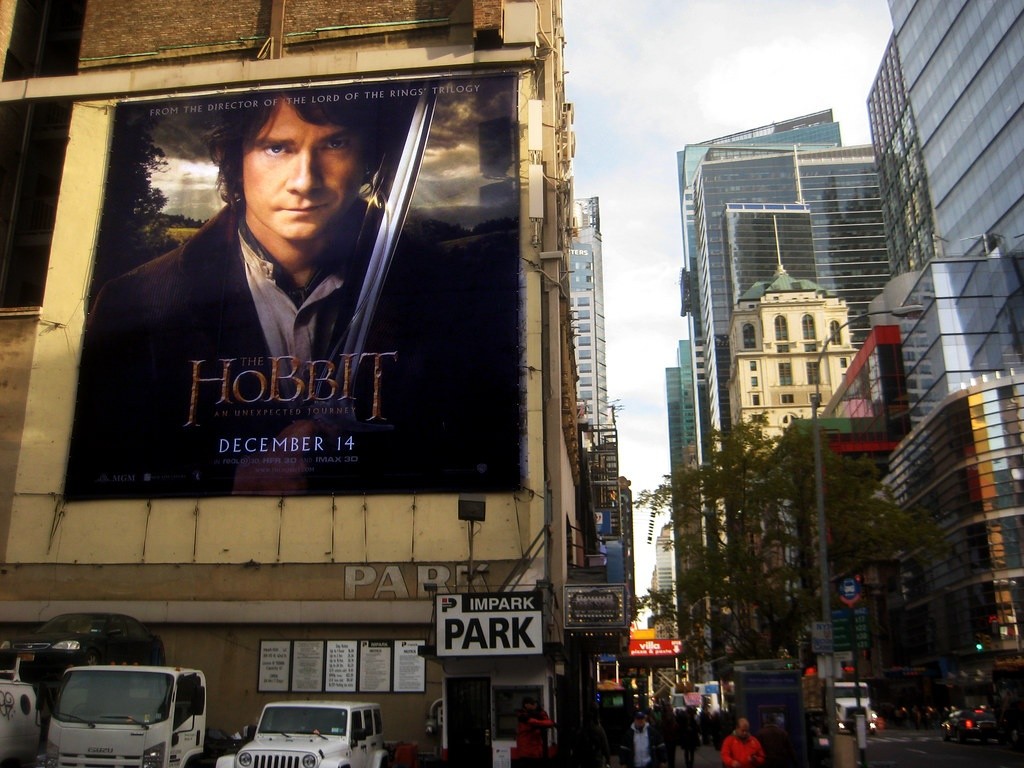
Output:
top-left (811, 304), bottom-right (923, 642)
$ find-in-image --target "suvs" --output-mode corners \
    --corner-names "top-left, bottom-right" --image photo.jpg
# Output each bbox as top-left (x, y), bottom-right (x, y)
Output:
top-left (216, 694), bottom-right (388, 768)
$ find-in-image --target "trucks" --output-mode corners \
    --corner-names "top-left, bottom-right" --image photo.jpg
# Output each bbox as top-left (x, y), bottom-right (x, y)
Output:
top-left (36, 665), bottom-right (256, 768)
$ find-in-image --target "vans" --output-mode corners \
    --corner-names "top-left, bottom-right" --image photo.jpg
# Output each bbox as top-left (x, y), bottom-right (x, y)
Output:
top-left (833, 682), bottom-right (876, 736)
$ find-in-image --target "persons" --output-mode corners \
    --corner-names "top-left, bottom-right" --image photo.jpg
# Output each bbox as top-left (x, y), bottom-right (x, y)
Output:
top-left (571, 702), bottom-right (798, 768)
top-left (719, 717), bottom-right (765, 768)
top-left (515, 695), bottom-right (559, 768)
top-left (617, 710), bottom-right (669, 767)
top-left (60, 81), bottom-right (521, 498)
top-left (877, 702), bottom-right (964, 733)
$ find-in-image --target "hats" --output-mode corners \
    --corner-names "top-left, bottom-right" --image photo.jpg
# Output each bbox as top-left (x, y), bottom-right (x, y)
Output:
top-left (635, 712), bottom-right (645, 720)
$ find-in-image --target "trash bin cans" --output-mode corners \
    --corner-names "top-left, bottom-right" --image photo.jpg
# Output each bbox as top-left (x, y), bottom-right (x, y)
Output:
top-left (834, 733), bottom-right (859, 768)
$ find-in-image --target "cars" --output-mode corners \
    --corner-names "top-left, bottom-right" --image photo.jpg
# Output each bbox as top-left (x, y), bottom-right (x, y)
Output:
top-left (0, 613), bottom-right (165, 676)
top-left (940, 707), bottom-right (997, 743)
